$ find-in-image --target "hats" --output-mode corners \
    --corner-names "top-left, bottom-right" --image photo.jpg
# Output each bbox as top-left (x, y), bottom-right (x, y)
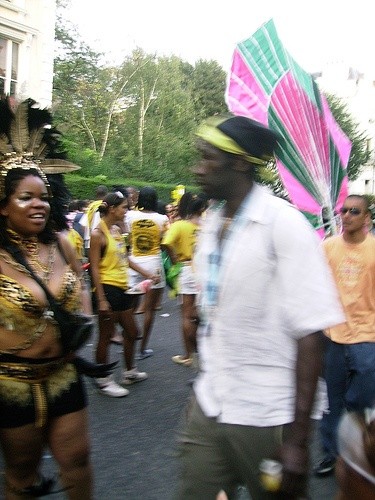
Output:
top-left (192, 113), bottom-right (280, 166)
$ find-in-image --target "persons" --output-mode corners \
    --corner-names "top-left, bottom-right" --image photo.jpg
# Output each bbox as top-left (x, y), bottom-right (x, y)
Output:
top-left (312, 195), bottom-right (375, 477)
top-left (66, 184), bottom-right (212, 397)
top-left (0, 165), bottom-right (96, 500)
top-left (177, 115), bottom-right (346, 500)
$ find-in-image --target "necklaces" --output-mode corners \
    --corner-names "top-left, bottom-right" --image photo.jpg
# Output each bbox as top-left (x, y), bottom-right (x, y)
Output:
top-left (26, 241), bottom-right (56, 276)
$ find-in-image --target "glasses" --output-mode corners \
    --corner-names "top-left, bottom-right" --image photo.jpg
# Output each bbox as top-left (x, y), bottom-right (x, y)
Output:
top-left (340, 207), bottom-right (361, 216)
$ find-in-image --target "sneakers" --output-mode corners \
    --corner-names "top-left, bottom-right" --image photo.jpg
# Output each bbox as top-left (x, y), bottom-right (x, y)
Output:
top-left (172, 354), bottom-right (194, 367)
top-left (134, 349), bottom-right (153, 360)
top-left (111, 336), bottom-right (123, 344)
top-left (94, 374), bottom-right (129, 398)
top-left (120, 367), bottom-right (148, 386)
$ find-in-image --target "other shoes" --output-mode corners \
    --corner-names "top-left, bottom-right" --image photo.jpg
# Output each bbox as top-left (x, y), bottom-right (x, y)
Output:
top-left (316, 458), bottom-right (335, 477)
top-left (136, 336), bottom-right (143, 340)
top-left (133, 306), bottom-right (162, 314)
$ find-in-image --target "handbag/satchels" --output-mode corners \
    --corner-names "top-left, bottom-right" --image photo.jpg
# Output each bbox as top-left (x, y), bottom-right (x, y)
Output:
top-left (58, 313), bottom-right (94, 354)
